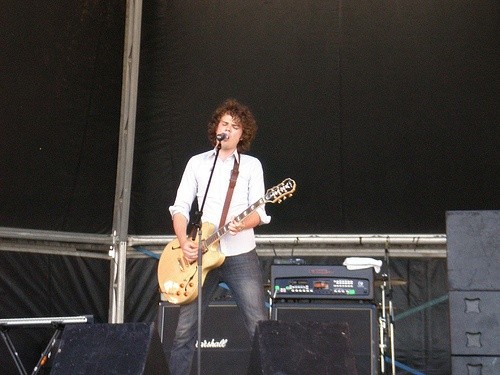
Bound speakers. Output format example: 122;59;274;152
247;319;357;375
159;301;270;375
51;323;171;375
271;304;379;375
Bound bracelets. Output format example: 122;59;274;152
239;220;246;232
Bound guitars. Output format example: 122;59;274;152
157;176;297;305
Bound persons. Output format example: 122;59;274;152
168;98;273;375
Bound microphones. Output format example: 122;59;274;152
217;133;227;142
